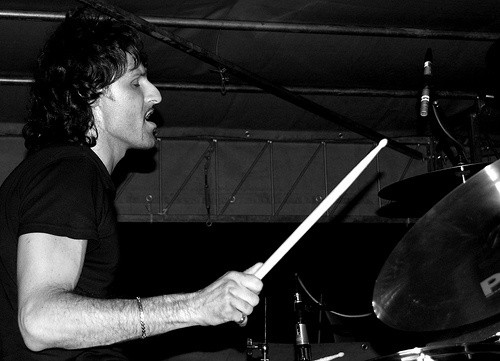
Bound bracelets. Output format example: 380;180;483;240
136;296;147;339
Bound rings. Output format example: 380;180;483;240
236;312;246;324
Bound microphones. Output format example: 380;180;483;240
420;48;434;117
293;292;312;361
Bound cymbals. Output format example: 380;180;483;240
371;158;500;332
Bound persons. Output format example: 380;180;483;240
0;9;263;361
